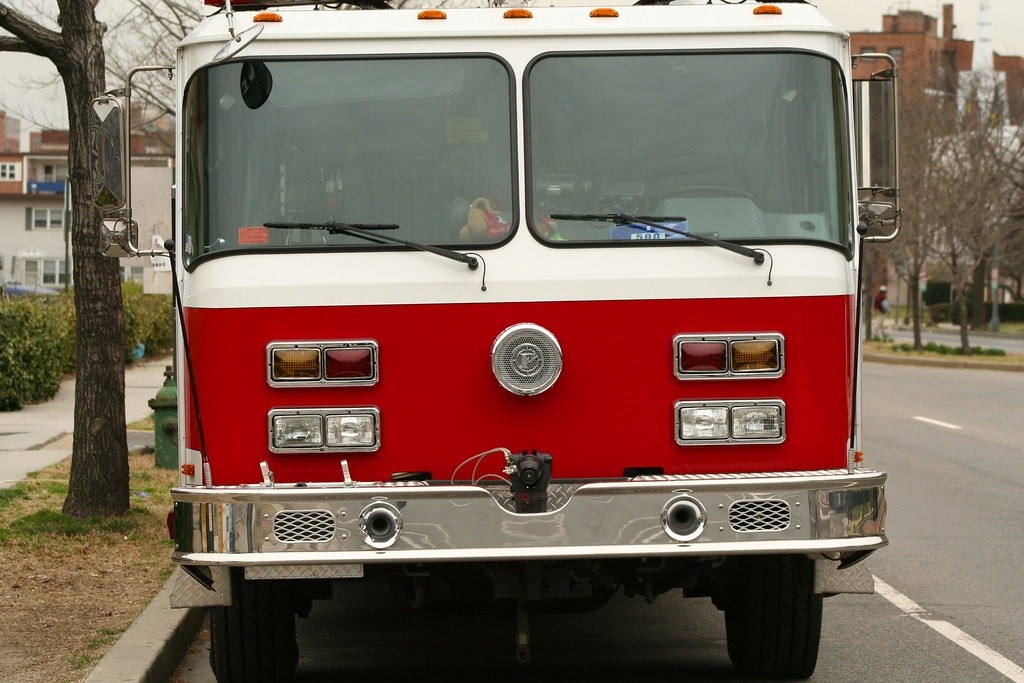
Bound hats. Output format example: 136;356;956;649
879;286;886;292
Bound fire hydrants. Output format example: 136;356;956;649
148;366;178;470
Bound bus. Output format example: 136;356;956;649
87;0;904;683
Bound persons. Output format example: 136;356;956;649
872;285;893;338
